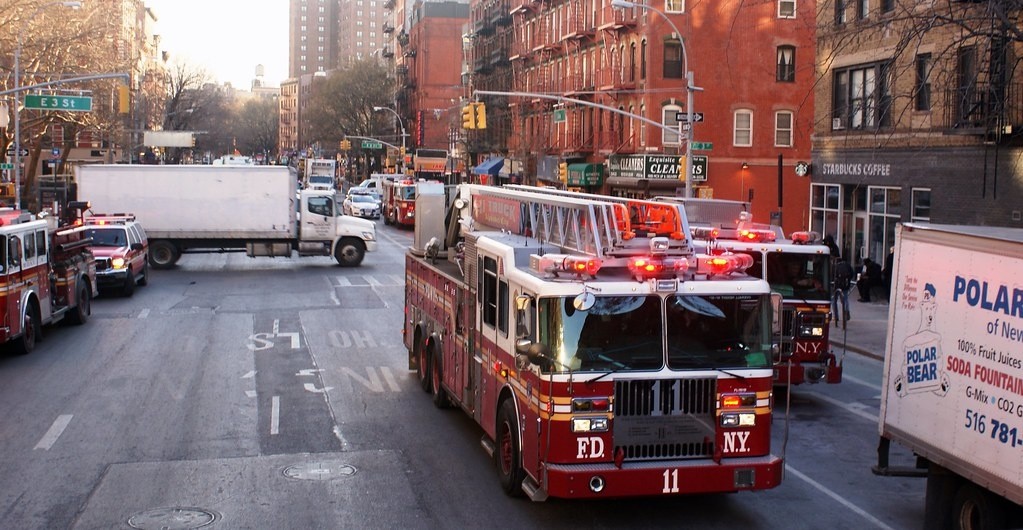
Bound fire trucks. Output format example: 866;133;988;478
0;208;99;354
403;182;843;502
382;178;416;231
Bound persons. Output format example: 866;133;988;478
828;249;853;321
857;258;881;302
822;235;840;258
881;246;894;301
672;305;709;341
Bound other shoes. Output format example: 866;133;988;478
858;297;870;302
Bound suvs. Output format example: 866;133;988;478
84;213;149;297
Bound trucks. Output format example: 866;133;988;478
361;174;403;197
871;223;1023;530
74;165;377;269
302;159;336;190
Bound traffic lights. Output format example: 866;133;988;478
556;162;567;184
401;147;405;156
462;106;476;129
679;155;687;184
340;141;352;150
476;105;486;129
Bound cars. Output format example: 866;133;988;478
343;187;382;219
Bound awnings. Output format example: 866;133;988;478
474;156;504;175
568;163;603;186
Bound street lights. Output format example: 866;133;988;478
14;3;81;211
374;107;406;175
611;0;693;200
